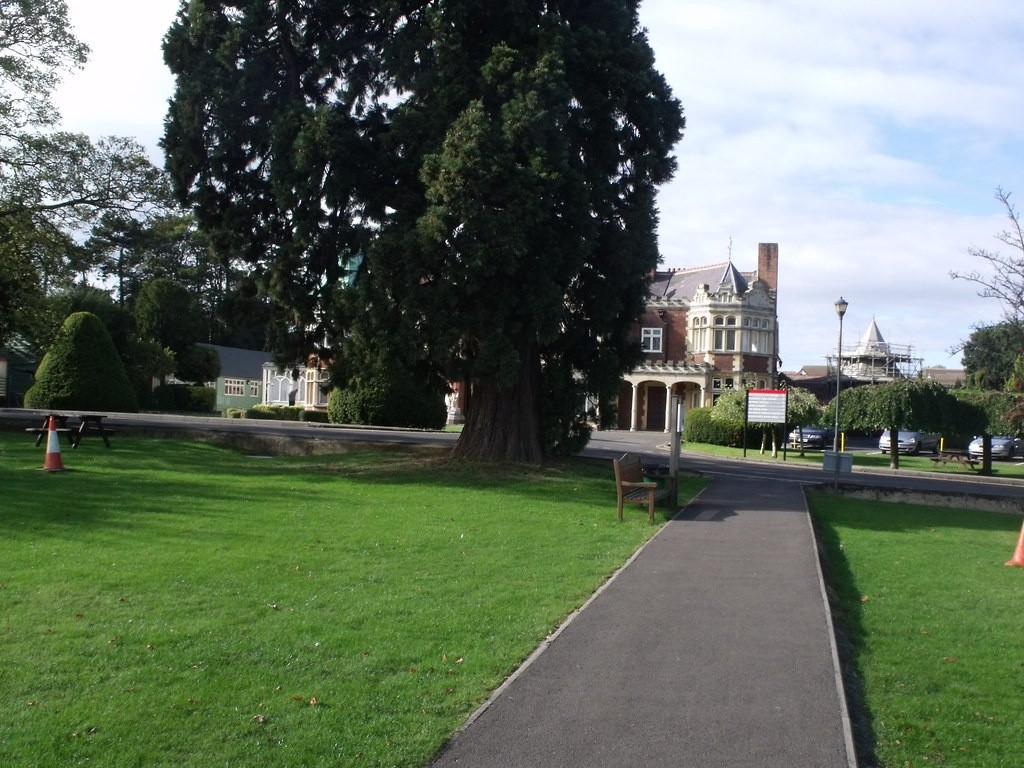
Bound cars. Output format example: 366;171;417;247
788;425;848;451
969;434;1024;462
879;427;945;456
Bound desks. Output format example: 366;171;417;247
34;413;111;449
932;451;974;472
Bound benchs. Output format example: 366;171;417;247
25;426;120;436
614;452;676;520
930;459;980;464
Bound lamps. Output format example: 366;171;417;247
659;309;665;317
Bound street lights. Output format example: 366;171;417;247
833;295;849;456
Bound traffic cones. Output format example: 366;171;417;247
42;416;65;471
1003;519;1024;568
